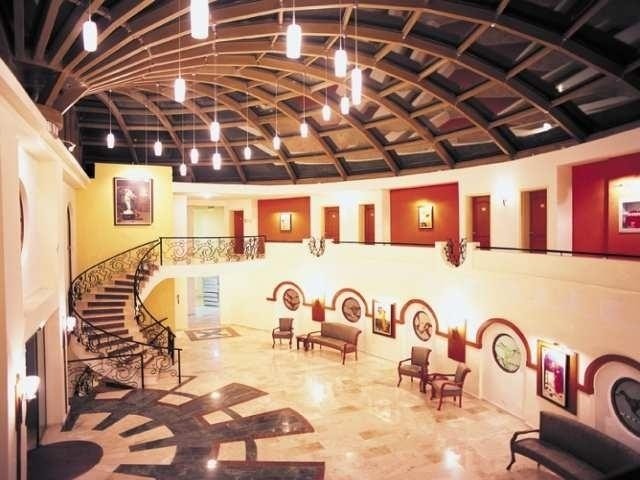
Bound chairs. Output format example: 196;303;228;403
272;317;294;349
397;345;432;392
430;360;472;411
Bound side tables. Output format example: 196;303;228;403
424;372;448;397
296;334;310;350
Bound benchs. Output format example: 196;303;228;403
307;320;362;365
506;411;640;479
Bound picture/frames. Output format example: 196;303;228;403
280;213;292;231
417;204;433;229
113;178;154;226
448;316;467;363
542;344;570;409
372;298;393;338
618;199;640;234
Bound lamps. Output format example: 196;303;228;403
341;81;350;116
334;7;346;76
211;34;221;143
287;3;302;60
301;75;308;137
174;3;185;102
323;60;330;121
213;146;222;169
108;91;114;148
274;84;280;150
17;374;42;402
154;85;161;156
352;5;362;103
180;101;187;177
194;3;209;39
85;5;97;52
191;85;198;163
245;89;250;159
67;316;77;333
